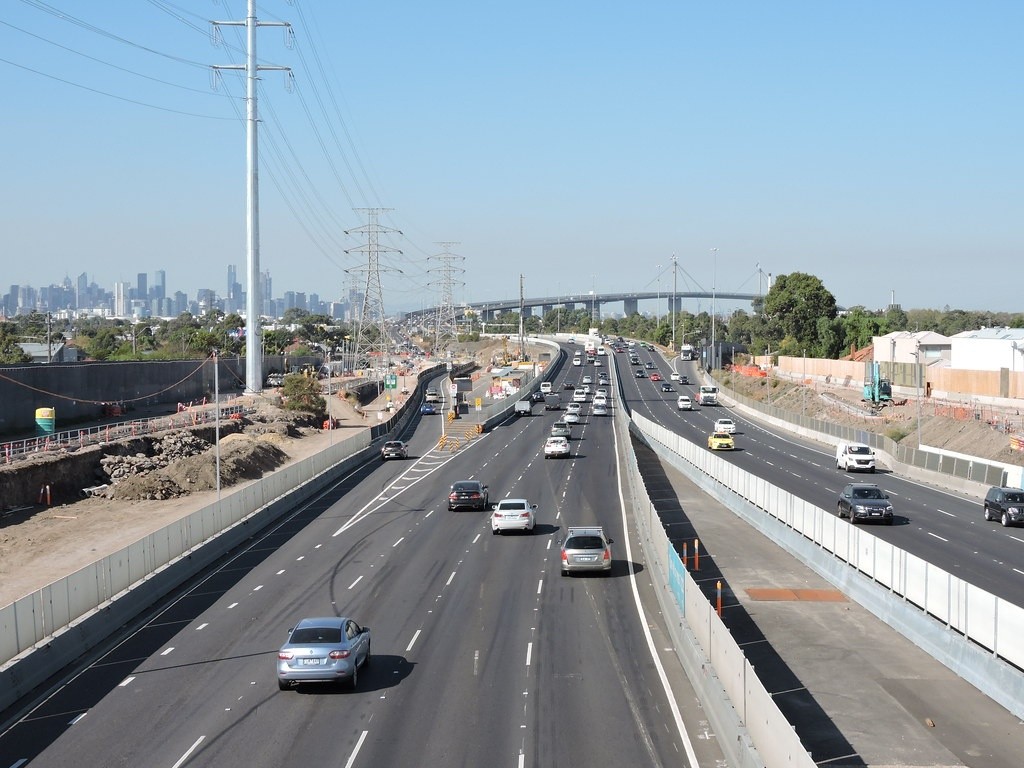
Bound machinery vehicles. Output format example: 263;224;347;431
862;361;892;409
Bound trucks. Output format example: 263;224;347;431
515;400;532;417
695;385;719;406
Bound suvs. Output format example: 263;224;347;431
551;422;572;438
837;483;893;525
558;525;615;576
425;387;439;402
544;436;571;459
983;486;1024;527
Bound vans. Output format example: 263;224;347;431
677;396;692;411
835;442;876;473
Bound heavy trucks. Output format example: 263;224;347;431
680;345;695;362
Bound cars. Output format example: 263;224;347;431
277;616;372;692
381;441;408;460
589;327;689;391
529;338;609;423
448;480;489;511
708;418;737;450
490;499;538;535
421;404;436;416
